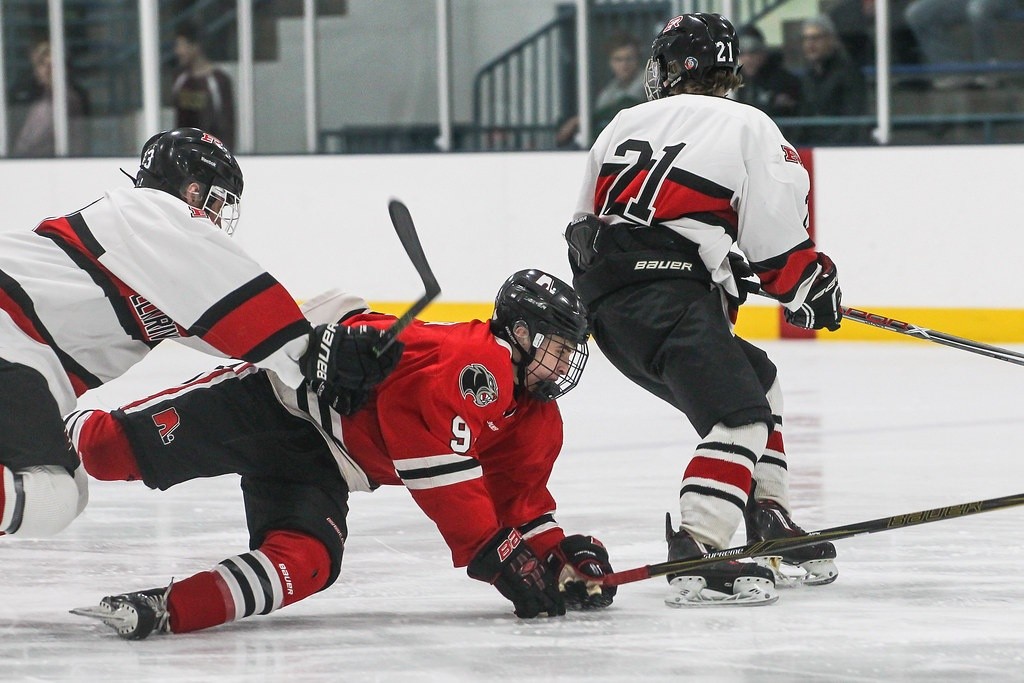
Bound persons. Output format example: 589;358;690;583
61;269;617;640
553;28;649;151
10;41;94;158
0;126;405;539
736;0;1007;146
161;20;240;156
566;12;843;609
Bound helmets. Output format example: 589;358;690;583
135;127;243;234
492;268;592;403
643;11;738;100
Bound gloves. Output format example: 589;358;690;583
543;534;617;610
467;525;566;618
299;321;403;415
782;256;844;330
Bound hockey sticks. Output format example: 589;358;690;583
370;197;445;361
600;492;1024;590
743;278;1024;369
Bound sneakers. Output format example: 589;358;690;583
665;511;779;606
744;477;837;588
70;575;177;641
